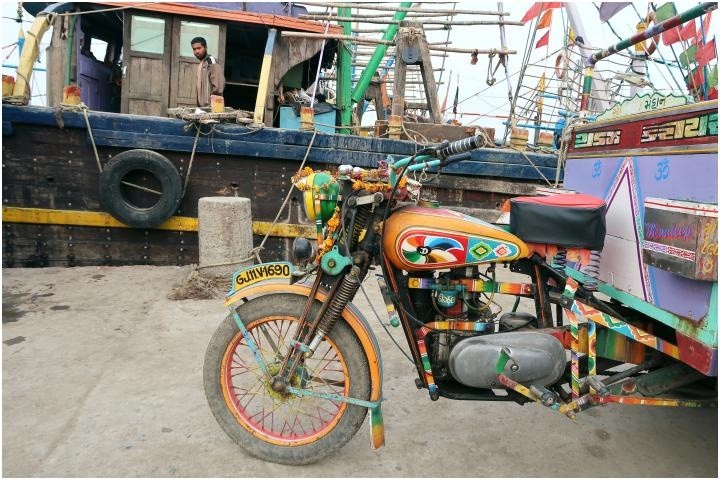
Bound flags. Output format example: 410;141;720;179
520;2;718;103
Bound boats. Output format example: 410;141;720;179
2;2;565;267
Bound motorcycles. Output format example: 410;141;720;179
200;2;718;467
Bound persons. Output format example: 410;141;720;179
191;37;227;112
